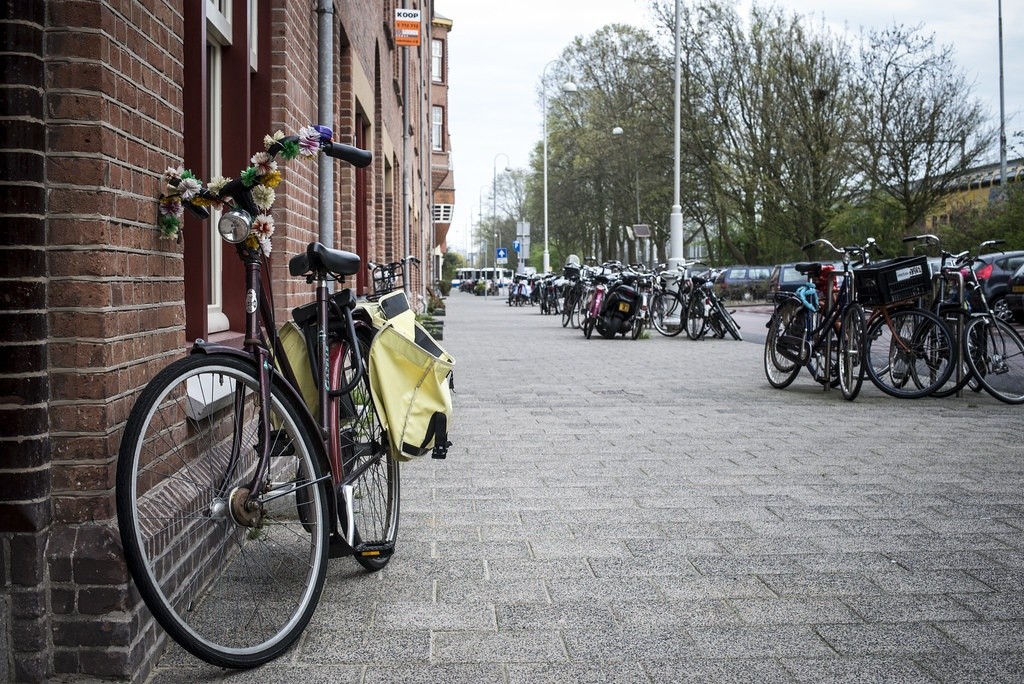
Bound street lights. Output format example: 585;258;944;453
541;58;578;275
477;153;512;299
612;109;641;223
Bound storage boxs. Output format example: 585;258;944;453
852;255;934;307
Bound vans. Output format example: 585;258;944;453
688;260;857;302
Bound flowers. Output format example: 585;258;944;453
157;126;321;258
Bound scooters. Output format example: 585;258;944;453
594;270;642;339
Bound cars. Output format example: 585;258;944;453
836;250;1024;325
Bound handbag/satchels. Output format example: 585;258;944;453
258;319;358;458
363;290;457;461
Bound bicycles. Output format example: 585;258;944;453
763;233;1024;405
506;255;742;342
116;125;424;669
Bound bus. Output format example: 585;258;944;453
452;267;513;288
